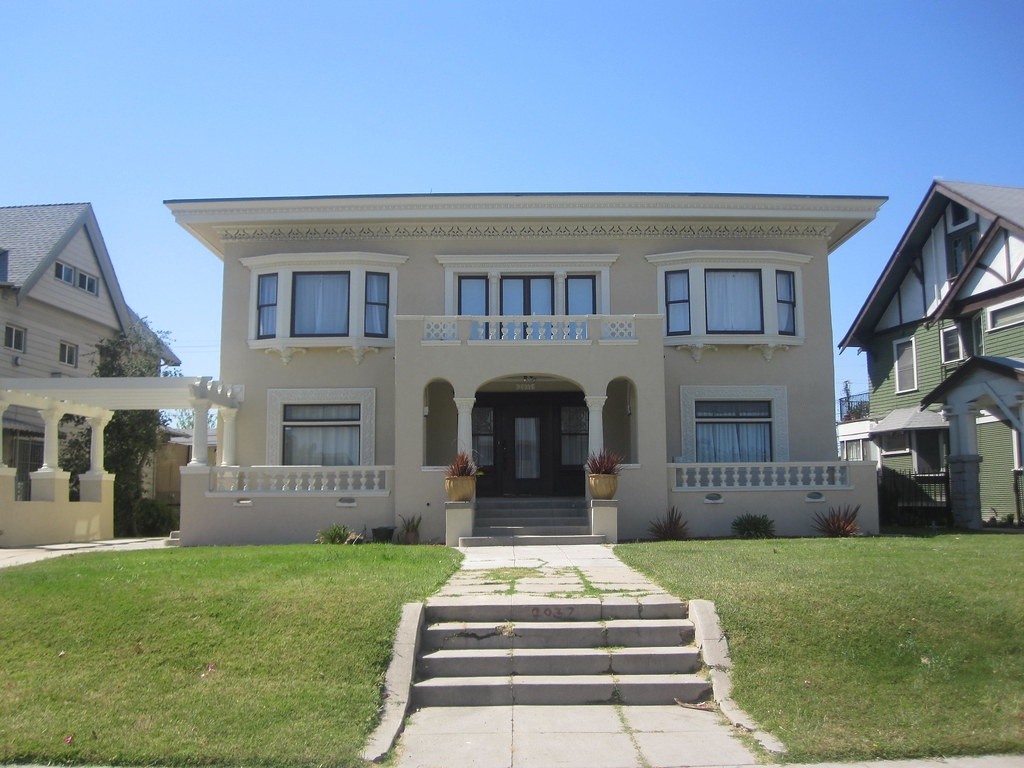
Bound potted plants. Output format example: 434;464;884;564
584;451;621;498
444;454;478;502
